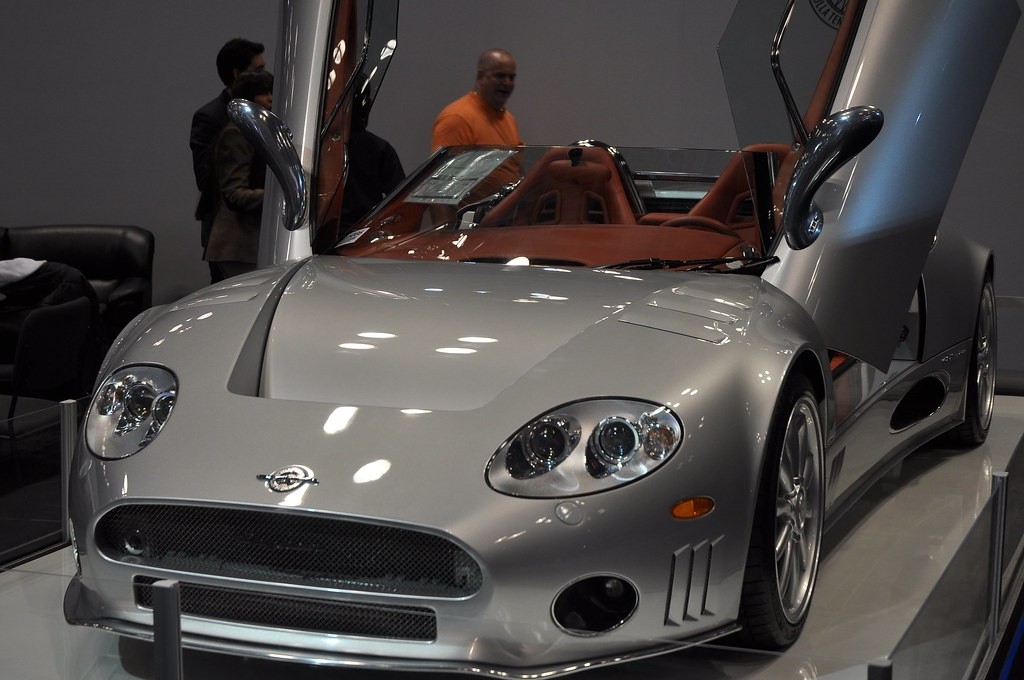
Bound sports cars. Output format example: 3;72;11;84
61;0;997;680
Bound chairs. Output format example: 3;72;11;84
0;224;154;456
480;147;646;228
687;144;796;251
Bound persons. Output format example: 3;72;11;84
311;72;406;255
204;64;274;279
189;38;267;286
431;49;526;226
